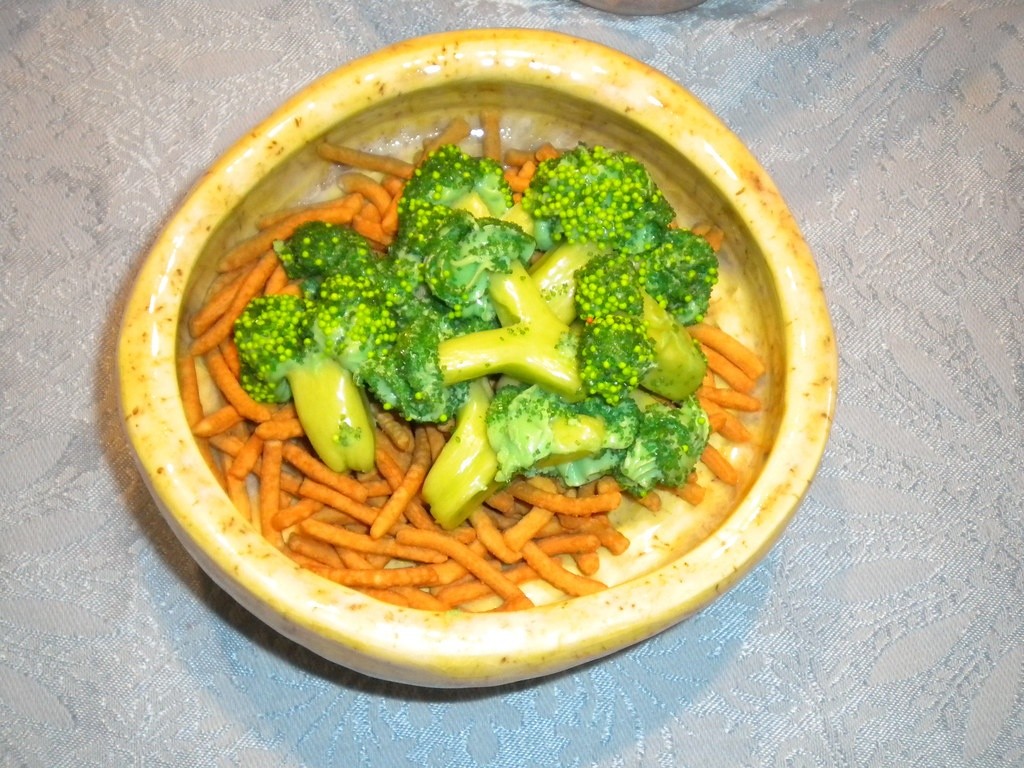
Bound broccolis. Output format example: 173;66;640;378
231;147;721;530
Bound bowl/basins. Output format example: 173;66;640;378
117;28;838;689
